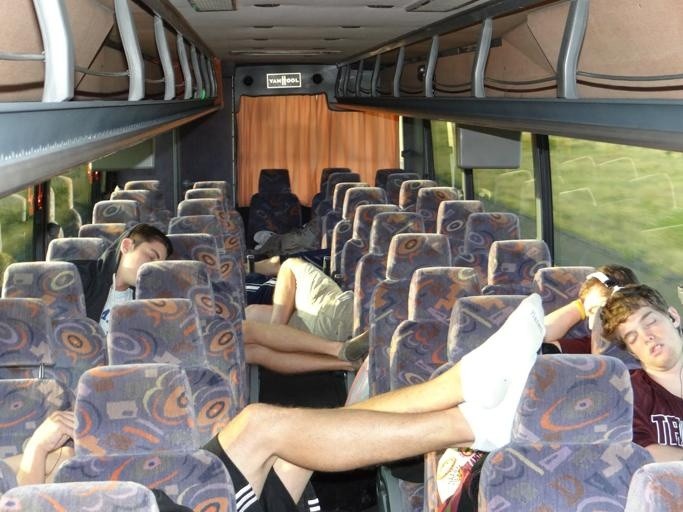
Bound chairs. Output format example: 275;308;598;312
0;166;259;511
311;167;682;511
245;166;303;262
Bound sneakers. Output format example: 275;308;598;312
336;331;369;362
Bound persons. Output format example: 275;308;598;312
435;262;640;512
599;282;683;464
1;292;547;512
